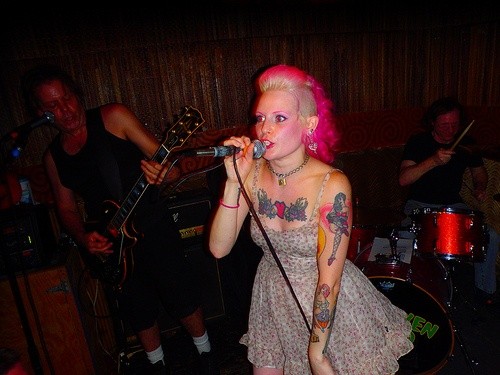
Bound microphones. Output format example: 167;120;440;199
179;140;264;158
0;110;54;143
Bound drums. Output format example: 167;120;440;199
341;223;379;262
354;243;459;375
408;206;495;261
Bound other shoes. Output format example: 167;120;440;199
192;346;219;375
150;360;172;375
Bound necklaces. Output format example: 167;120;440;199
266;153;309;186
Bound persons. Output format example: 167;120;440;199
21;64;219;375
209;64;414;375
399;96;500;307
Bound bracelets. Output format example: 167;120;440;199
220;199;239;208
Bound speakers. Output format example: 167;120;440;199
112;187;226;346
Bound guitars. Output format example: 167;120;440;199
78;105;207;293
